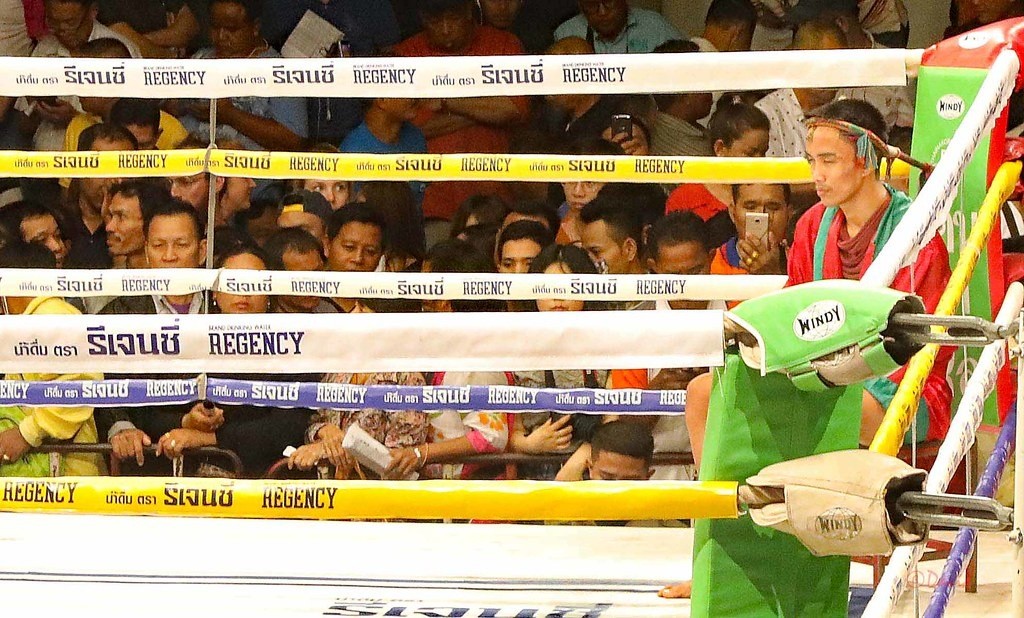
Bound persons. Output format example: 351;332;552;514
0;0;1024;528
662;99;956;599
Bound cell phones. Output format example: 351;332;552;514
611;114;633;141
745;212;769;251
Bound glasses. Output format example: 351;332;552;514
163;170;209;193
44;1;93;39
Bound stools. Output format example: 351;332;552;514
852;436;979;591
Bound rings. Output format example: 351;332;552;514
751;251;758;260
3;454;10;461
746;258;752;266
210;426;213;430
164;432;170;438
171;440;175;447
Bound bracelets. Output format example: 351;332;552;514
414;447;422;465
421;443;428;468
